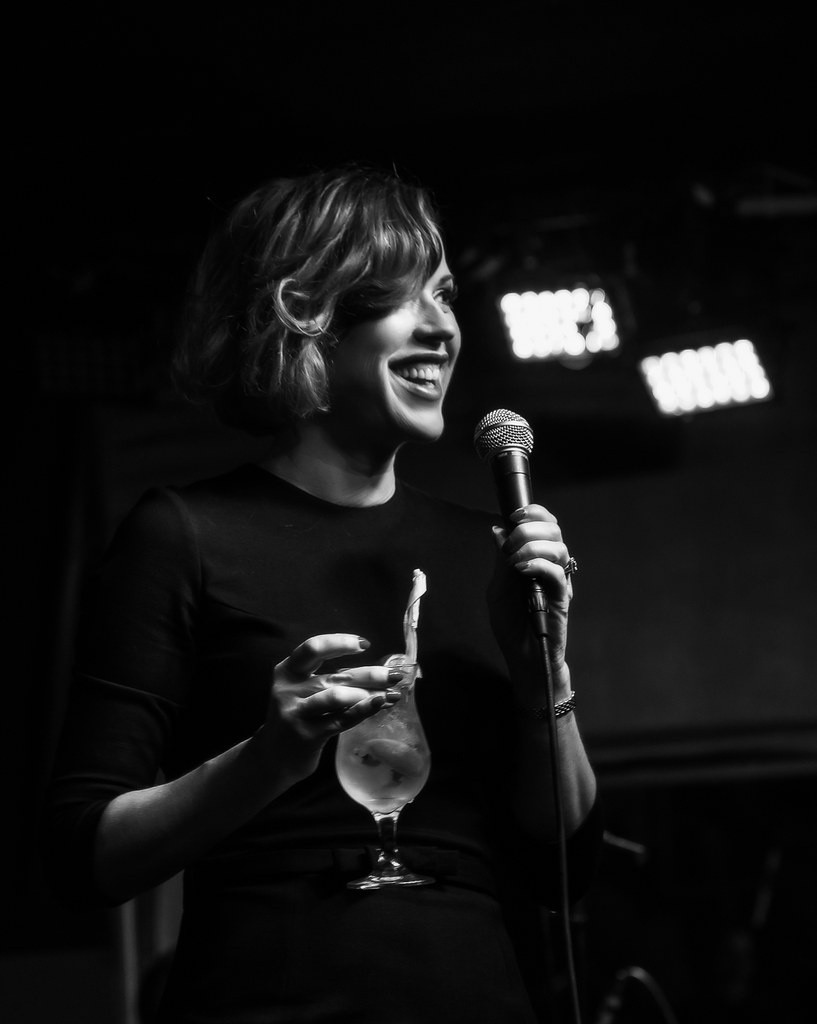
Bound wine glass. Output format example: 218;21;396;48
335;667;432;890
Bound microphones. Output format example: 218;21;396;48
474;410;551;638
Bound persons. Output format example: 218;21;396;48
42;165;598;1024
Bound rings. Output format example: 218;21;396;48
564;556;578;576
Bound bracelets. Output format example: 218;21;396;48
554;690;578;719
